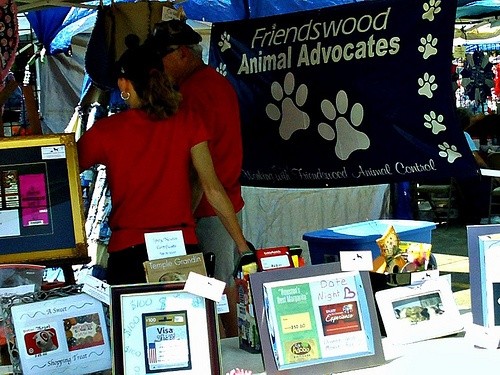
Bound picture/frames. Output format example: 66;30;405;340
0;132;88;265
375;276;465;348
467;224;500;326
0;283;113;375
248;261;386;375
109;281;224;375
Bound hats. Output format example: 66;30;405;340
144;20;202;45
108;44;164;80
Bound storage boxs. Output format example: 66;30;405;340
235;277;262;354
302;219;438;265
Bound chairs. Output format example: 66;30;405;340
411;179;454;230
475;168;500;223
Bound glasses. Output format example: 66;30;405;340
164;46;191;55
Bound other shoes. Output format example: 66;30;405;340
480;218;493;224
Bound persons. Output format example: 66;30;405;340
76;45;254;284
451;106;487;226
149;18;245;338
0;73;22;122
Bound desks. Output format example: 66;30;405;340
198;183;391;288
